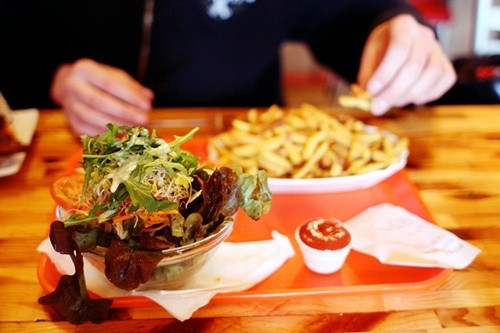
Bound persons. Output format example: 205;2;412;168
1;0;460;140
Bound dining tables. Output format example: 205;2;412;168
0;102;500;331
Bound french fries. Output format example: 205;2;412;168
209;84;410;177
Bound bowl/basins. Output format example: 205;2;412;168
294;218;352;274
56;196;240;292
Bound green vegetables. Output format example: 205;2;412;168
37;121;273;326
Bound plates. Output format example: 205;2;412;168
265;125;409;194
1;107;41;177
